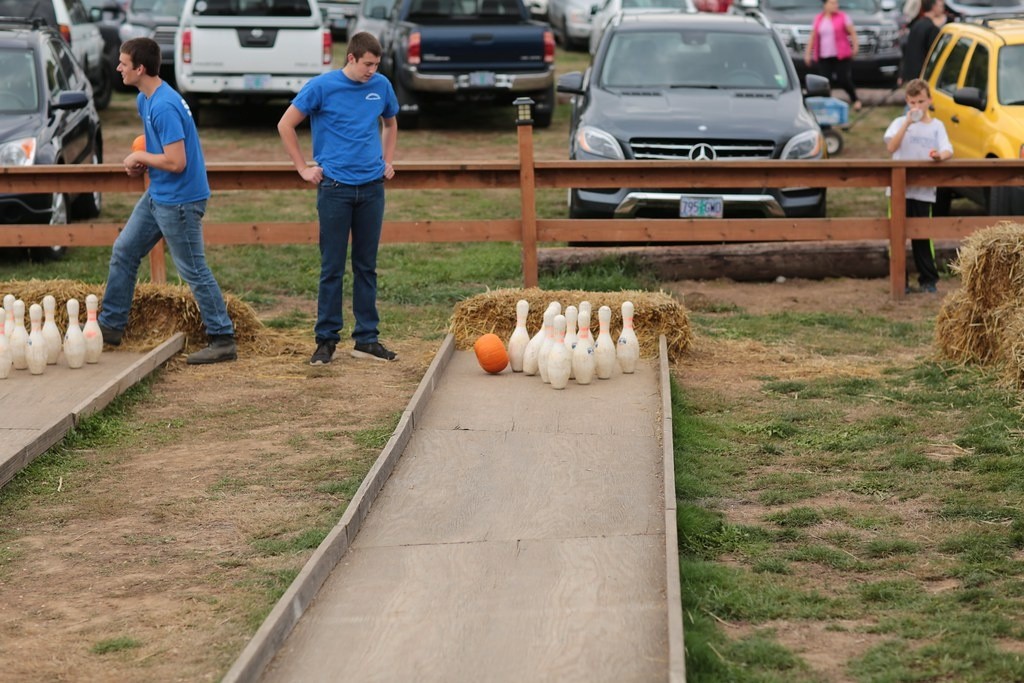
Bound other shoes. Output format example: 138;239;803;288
903;284;935;295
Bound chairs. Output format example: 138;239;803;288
607;38;670;82
470;0;503;19
410;0;447;19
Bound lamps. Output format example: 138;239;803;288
512;97;535;125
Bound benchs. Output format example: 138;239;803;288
198;0;304;15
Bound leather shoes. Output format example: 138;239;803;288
186;339;238;364
65;320;123;346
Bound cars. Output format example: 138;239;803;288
549;1;605;44
318;1;392;46
904;0;1024;33
0;1;113;113
84;0;183;89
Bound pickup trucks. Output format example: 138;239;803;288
379;1;555;131
173;0;333;125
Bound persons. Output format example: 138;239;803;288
898;0;946;86
98;37;238;365
805;0;864;113
882;80;954;292
278;31;399;363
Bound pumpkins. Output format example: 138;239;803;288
473;333;508;373
132;135;146;152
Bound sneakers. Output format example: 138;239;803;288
309;340;340;365
351;340;398;361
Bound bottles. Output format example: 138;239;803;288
910;109;924;122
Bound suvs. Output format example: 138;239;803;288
919;12;1024;216
730;1;902;90
557;8;830;247
0;16;102;260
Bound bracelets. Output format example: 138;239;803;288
299;166;308;173
